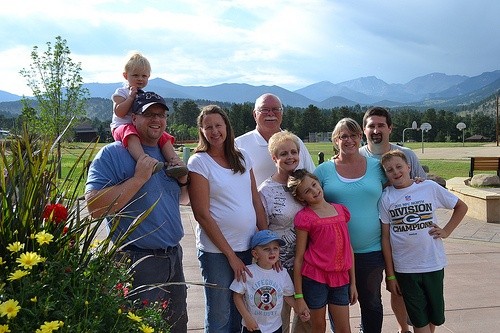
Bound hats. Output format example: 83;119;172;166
133;91;169;115
251;229;286;249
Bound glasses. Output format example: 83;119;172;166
259;107;283;114
142;112;167;118
340;134;359;140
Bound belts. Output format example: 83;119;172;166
124;245;180;255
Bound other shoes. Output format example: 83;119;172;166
165;165;188;178
153;162;164;175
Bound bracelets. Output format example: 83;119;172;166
180;179;189;187
294;294;303;298
388;276;395;280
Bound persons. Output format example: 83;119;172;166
256;129;308;333
287;169;358;333
110;53;188;179
312;118;424;333
377;149;469;333
187;105;269;333
85;92;191;333
234;92;315;333
358;106;428;333
229;230;310;333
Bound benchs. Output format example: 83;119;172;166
469;157;500;178
